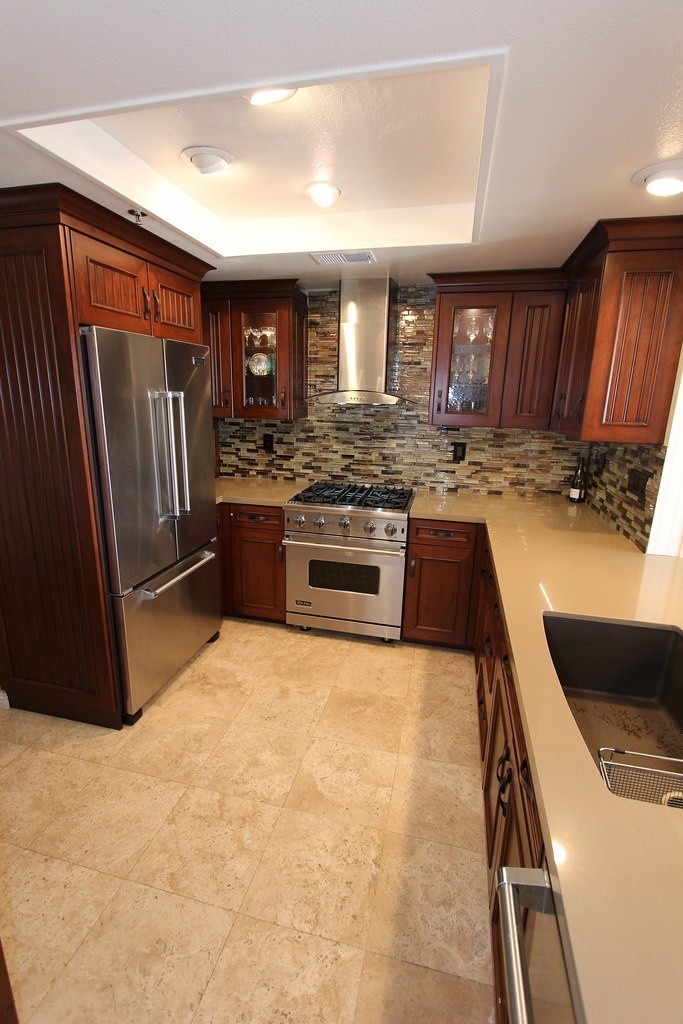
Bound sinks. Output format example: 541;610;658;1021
542;609;683;811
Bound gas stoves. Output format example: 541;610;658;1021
289;480;415;513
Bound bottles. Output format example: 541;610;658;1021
570;457;586;503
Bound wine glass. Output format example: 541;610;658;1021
244;326;252;346
251;328;263;347
245;356;250;376
261;326;276;347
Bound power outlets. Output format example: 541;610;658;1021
263;433;274;451
627;468;647;496
453;442;467;461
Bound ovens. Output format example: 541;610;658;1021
281;530;406;643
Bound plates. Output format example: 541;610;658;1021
249;353;268;376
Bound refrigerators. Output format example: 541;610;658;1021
78;325;223;726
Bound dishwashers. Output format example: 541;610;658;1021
495;849;584;1024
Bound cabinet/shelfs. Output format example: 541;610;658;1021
472;525;507;762
482;655;544;929
425;267;565;431
70;230;204;344
228;504;286;624
548;214;683;445
401;518;478;651
200;278;308;421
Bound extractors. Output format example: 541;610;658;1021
302;277;417;408
